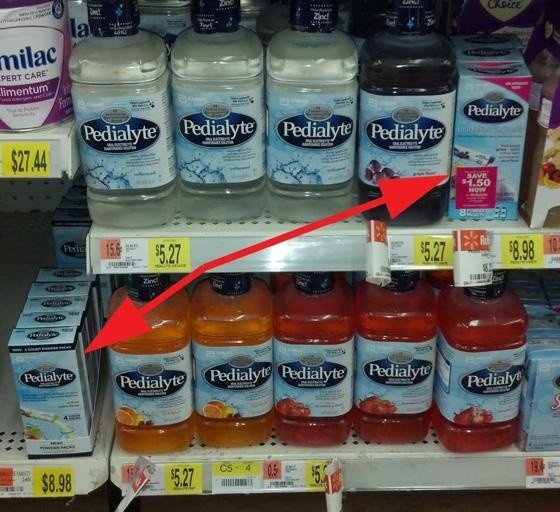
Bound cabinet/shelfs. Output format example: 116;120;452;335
0;122;560;500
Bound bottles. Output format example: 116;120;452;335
68;0;457;229
518;40;560;229
106;271;526;454
